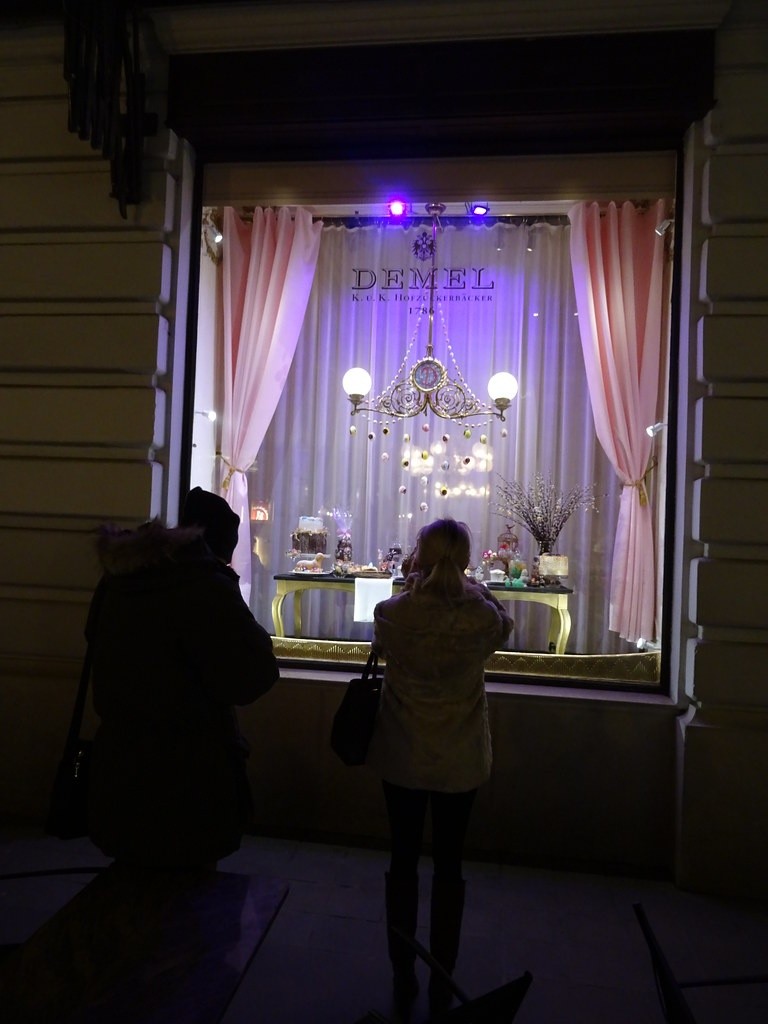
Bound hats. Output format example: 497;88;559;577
181;486;240;553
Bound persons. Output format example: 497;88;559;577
79;487;281;1019
371;516;514;1020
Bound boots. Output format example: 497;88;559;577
384;871;420;1004
427;874;466;1014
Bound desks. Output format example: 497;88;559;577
272;572;573;655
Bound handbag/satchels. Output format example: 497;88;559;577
42;745;101;839
329;648;383;768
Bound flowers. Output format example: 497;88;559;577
485;471;610;554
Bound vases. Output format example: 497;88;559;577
533;554;569;584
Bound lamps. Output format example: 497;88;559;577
341;202;518;512
